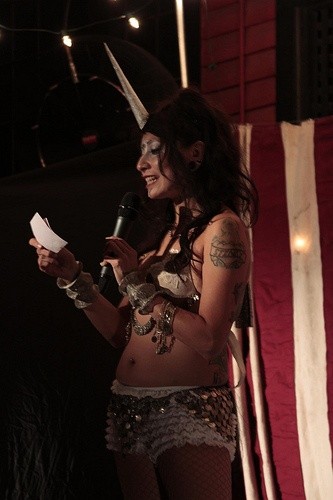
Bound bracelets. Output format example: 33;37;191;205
118;270;177;354
55;261;99;308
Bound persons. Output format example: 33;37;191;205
27;87;258;500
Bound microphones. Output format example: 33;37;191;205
94;194;141;296
